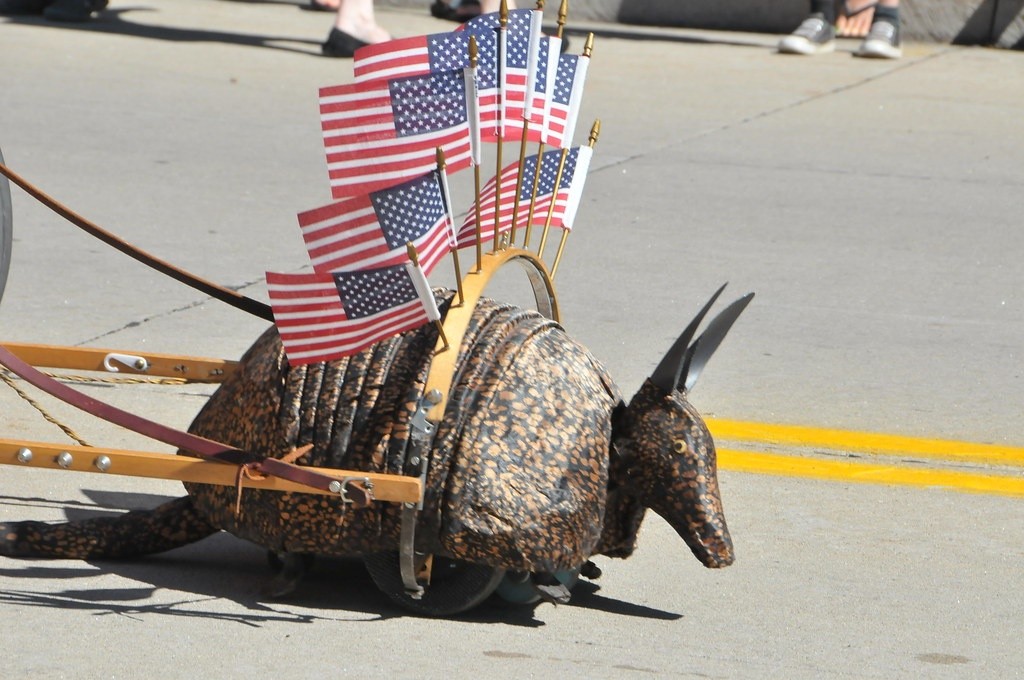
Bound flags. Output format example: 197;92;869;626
482;36;562;144
450;146;596;252
297;171;458;277
548;52;591;148
355;30;505;142
452;9;543;120
268;265;438;369
318;70;480;199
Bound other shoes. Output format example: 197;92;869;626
327;28;397;55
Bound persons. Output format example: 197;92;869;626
431;1;568;52
322;0;395;57
779;0;901;60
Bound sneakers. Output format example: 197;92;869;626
862;18;902;57
778;13;834;54
